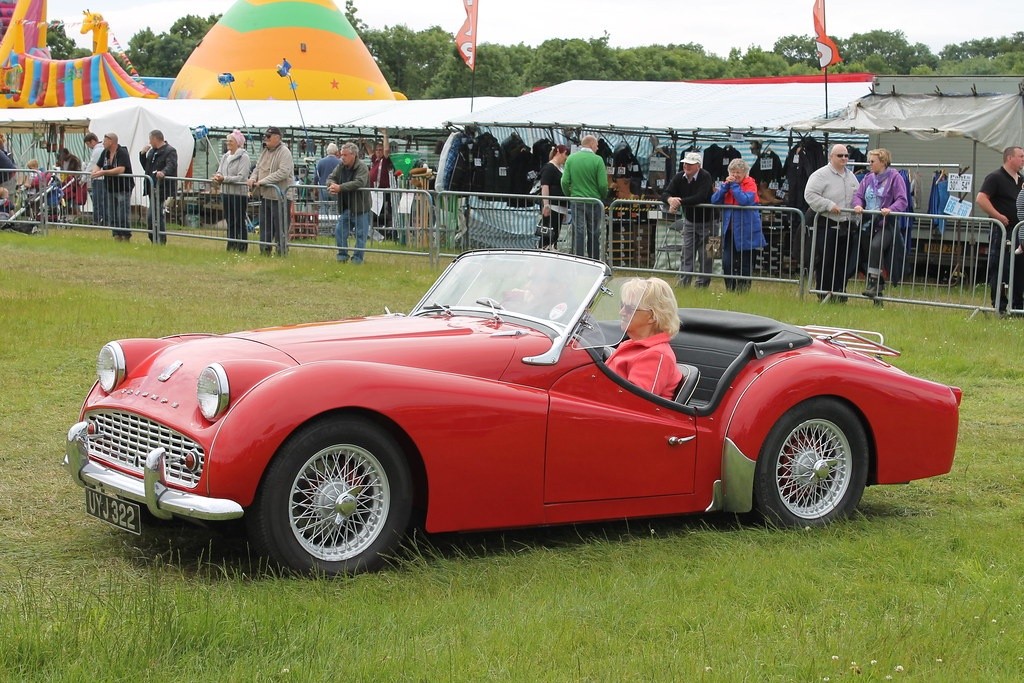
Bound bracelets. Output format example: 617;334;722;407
544;204;549;208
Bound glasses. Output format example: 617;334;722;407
104;134;112;140
620;301;647;311
833;153;849;158
264;134;271;139
868;159;880;163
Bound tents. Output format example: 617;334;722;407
88;80;874;168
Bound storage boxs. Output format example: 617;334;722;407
603;195;666;266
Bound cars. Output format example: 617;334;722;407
63;246;958;583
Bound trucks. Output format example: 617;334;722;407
865;72;1024;284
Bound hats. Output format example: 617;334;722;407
679;152;702;165
261;126;282;137
231;129;245;146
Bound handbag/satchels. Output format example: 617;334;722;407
529;165;556;195
535;213;555;239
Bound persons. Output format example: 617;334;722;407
0;126;397;264
540;136;1024;317
604;278;682;401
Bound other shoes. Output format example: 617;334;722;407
862;276;885;308
114;234;130;243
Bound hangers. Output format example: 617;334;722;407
466;121;805;161
856;163;948;185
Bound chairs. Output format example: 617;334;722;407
599;321;750;403
288;196;319;240
651;218;683;274
